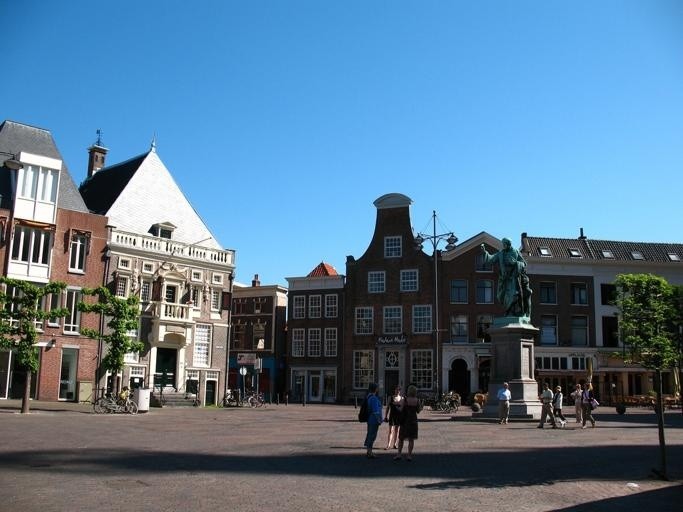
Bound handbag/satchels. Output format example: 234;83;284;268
589;399;599;409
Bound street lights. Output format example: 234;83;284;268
411;209;460;413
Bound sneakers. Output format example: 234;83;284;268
363;442;413;462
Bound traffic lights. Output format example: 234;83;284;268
220;292;231;309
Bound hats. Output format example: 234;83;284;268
554;386;561;391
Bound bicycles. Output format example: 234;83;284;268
415;388;461;414
224;387;264;408
91;385;141;417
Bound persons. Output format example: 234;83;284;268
554;386;568;424
482;238;527;316
497;382;511;424
364;382;383;458
392;385;420;461
384;385;403;449
571;384;584;424
537;383;557;429
581;383;597;429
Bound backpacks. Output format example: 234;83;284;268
359;393;373;422
